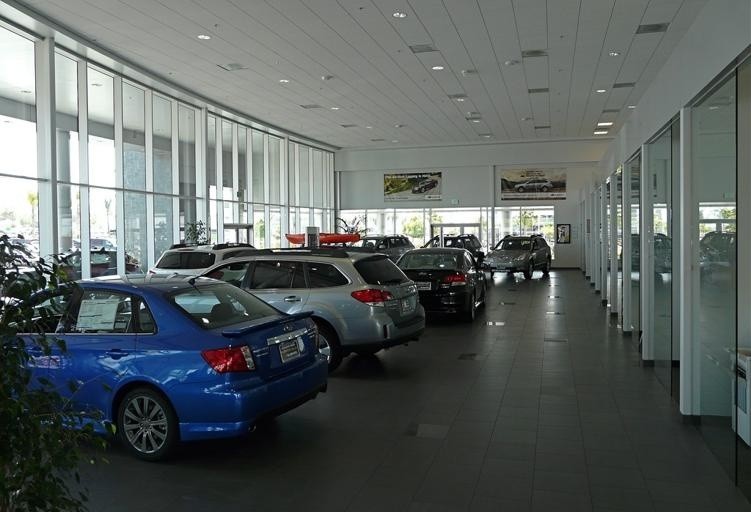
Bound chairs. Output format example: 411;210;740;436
209;304;233;321
127;309;154;335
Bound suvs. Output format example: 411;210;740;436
44;237;144;286
515;178;553;192
0;238;40;269
147;242;426;372
412;179;438;193
346;234;551;279
600;232;736;293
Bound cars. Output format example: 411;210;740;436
396;247;488;323
11;273;329;461
